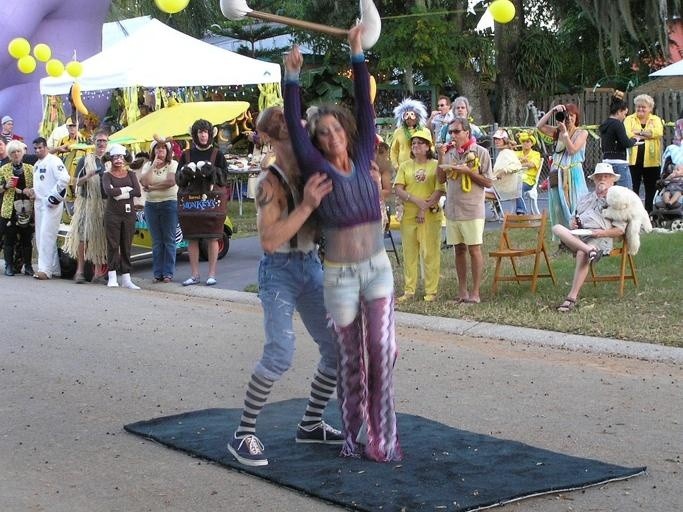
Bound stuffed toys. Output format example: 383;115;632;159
602;185;652;254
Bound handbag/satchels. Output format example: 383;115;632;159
550;170;558;187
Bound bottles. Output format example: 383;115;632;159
575;209;583;229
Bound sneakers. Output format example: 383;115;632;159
4;263;217;290
397;294;413;302
423;294;437;301
226;430;268;466
295;420;345;444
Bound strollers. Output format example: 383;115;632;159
648;144;682;232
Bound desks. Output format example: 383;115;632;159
226;157;263;215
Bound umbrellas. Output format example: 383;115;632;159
648;59;683;77
109;99;250;144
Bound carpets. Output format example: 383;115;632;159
124;397;649;512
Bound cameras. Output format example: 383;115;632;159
555;109;567;122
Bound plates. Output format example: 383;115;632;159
438;163;467;170
569;229;593;236
633;141;645;146
248;168;262;172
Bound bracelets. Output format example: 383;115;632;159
562;132;568;137
639;132;643;135
406;194;409;201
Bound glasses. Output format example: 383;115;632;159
437;104;444;107
448;129;462;135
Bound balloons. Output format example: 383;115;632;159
8;37;81;77
488;0;515;24
155;0;190;14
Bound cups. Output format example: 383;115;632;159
11;176;19;188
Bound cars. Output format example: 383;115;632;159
56;146;233;277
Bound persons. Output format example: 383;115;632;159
370;134;392;235
394;129;447;304
552;163;626;312
426;96;543;217
437;118;493;303
390;97;432;169
598;95;647;189
623;94;663;215
227;107;347;468
536;103;589;258
282;21;402;464
1;117;227;289
663;162;683;208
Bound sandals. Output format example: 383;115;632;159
556;297;577;314
588;246;604;264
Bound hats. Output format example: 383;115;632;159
150;134;172;151
66;117;79;126
519;129;537;145
587;162;621;183
101;144;132;164
2;115;14;124
410;128;433;146
492;130;508;144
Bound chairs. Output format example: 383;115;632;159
523;157;545;215
383;205;401;265
573;232;639;297
488;207;556;294
487;171;528;220
